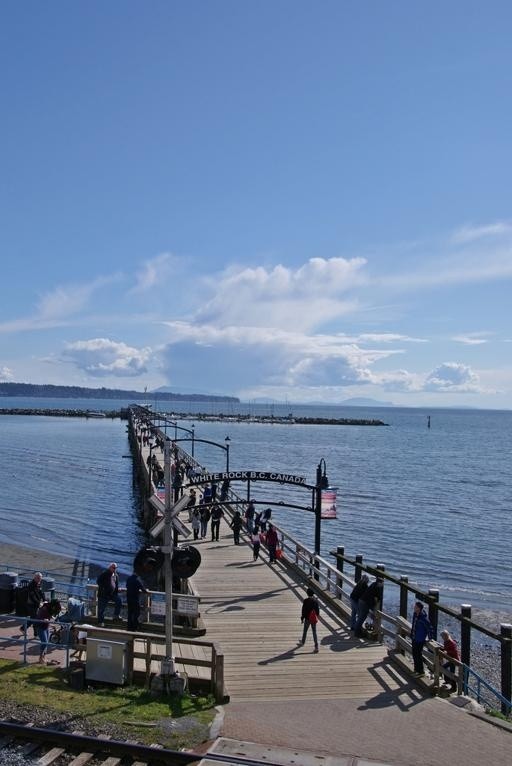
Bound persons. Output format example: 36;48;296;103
94;562;123;628
34;598;62;664
131;407;280;564
125;569;152;633
439;630;459;693
298;587;320;653
19;572;48;640
350;574;369;632
354;577;384;638
407;602;434;680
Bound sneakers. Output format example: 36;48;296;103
409;671;425;679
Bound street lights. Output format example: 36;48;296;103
158;412;177;443
314;458;329;581
224;436;231;472
191;423;196;457
148;434;155;490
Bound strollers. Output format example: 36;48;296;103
40;598;85;627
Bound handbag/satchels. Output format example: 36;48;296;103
259;534;266;544
276;549;283;559
309;610;318;624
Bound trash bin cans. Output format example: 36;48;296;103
40;576;57;603
16;578;33;617
0;571;18;614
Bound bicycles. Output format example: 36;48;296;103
49;618;79;645
